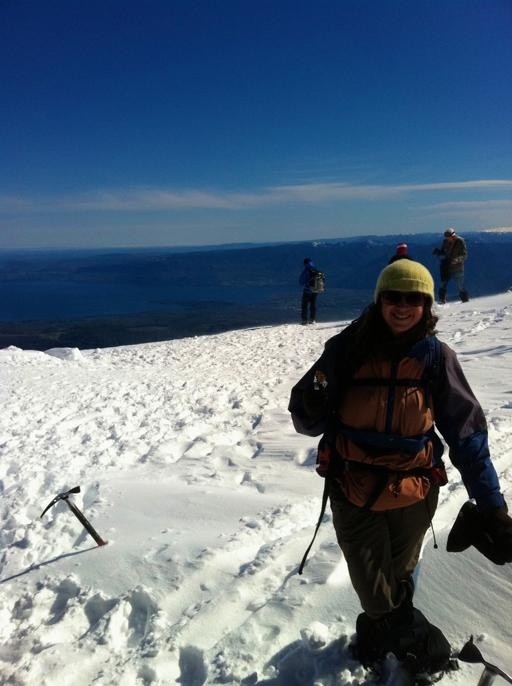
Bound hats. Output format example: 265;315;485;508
374;259;435;304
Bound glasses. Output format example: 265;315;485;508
381;291;425;306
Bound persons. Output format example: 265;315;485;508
431;228;469;304
388;242;413;266
298;257;317;326
288;258;510;686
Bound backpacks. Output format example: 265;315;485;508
304;268;325;294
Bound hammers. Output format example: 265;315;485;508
39;486;107;547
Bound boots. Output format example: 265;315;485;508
438;299;446;304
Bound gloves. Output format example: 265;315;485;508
296;380;333;419
447;496;512;565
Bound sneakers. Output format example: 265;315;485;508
301;320;316;325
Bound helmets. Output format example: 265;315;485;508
444;228;456;238
397;243;407;256
